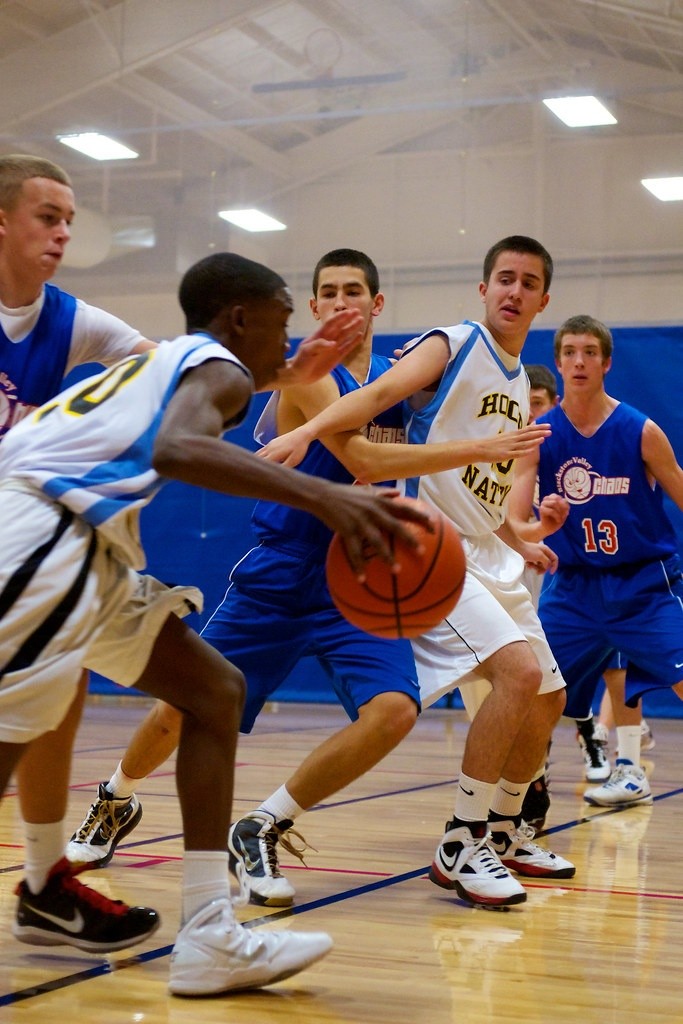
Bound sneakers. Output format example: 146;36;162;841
428;820;527;908
489;817;576;878
227;810;318;907
615;723;654;754
583;763;653;807
66;780;142;869
167;899;333;994
575;726;607;748
13;856;161;954
580;741;612;781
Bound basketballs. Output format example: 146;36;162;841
323;495;468;642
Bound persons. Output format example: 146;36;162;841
507;314;683;831
67;248;420;908
0;157;366;956
255;236;576;907
2;252;433;999
